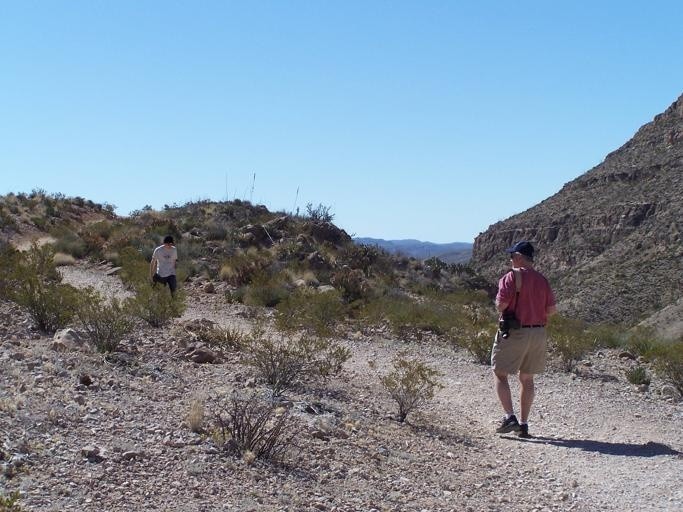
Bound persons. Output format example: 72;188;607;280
148;235;180;297
488;240;559;440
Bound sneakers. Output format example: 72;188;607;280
514;424;528;437
496;415;519;433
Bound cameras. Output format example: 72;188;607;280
498;310;520;339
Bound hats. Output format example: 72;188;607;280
506;241;533;257
163;235;174;244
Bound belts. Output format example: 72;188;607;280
521;325;546;327
